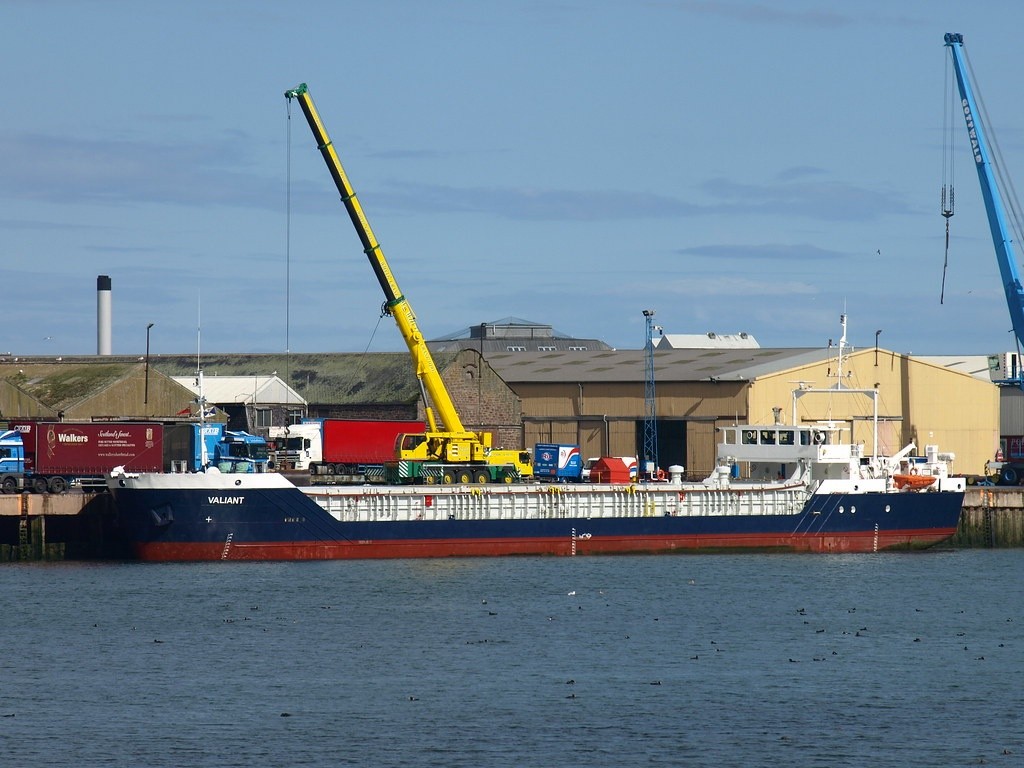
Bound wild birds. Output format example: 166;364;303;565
568;589;575;596
56;356;63;361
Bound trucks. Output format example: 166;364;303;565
165;421;276;473
533;442;585;484
0;419;164;495
580;456;637;483
977;459;1024;487
274;417;426;476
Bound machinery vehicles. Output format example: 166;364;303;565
938;32;1024;486
281;81;536;484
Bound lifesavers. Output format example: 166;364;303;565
657;470;665;481
747;432;753;440
910;468;918;475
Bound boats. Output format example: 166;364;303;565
66;316;968;566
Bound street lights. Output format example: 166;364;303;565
144;323;155;404
